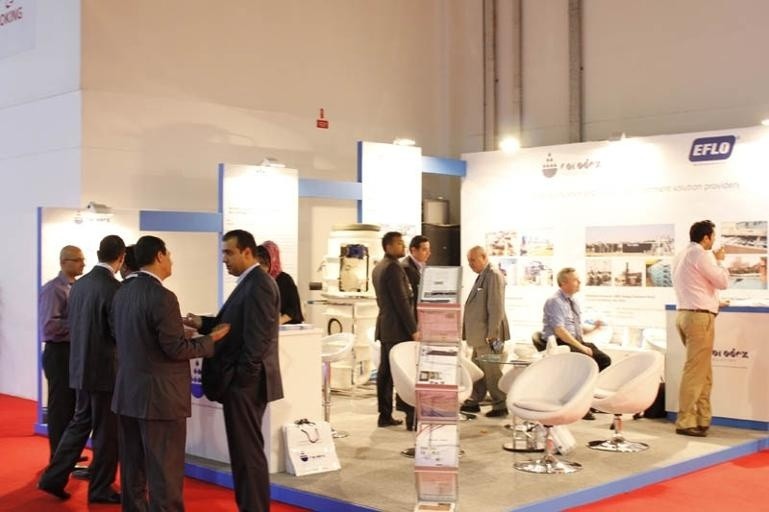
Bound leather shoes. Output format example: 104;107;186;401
460;405;480;413
407;426;416;431
698;425;709;431
582;412;595;420
590;408;604;413
90;493;121;503
378;417;403;427
485;408;508;417
73;469;91;478
78;457;89;462
38;477;72;500
72;465;88;471
675;428;705;436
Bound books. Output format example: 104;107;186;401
417;471;458;502
415;424;455;466
420;312;459;343
417;345;458;387
420;266;459;305
419;391;456;418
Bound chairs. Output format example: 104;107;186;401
639;326;667;383
504;351;600;474
586;349;666;454
497;344;571;455
321;332;355;440
387;340;475;459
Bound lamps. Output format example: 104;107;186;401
608;130;627;143
86;202;114;219
260;157;288;169
393;138;416;147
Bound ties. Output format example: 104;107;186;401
567;298;583;344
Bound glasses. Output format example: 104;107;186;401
63;257;85;262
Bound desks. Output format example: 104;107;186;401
667;302;769;431
475;352;530;365
185;325;322;476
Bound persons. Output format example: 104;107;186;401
109;235;231;512
670;219;732;438
461;244;511;417
370;231;420;430
542;268;612;421
251;241;304;326
117;243;144;279
36;235;128;505
179;229;285;512
399;235;431;327
40;245;90;463
